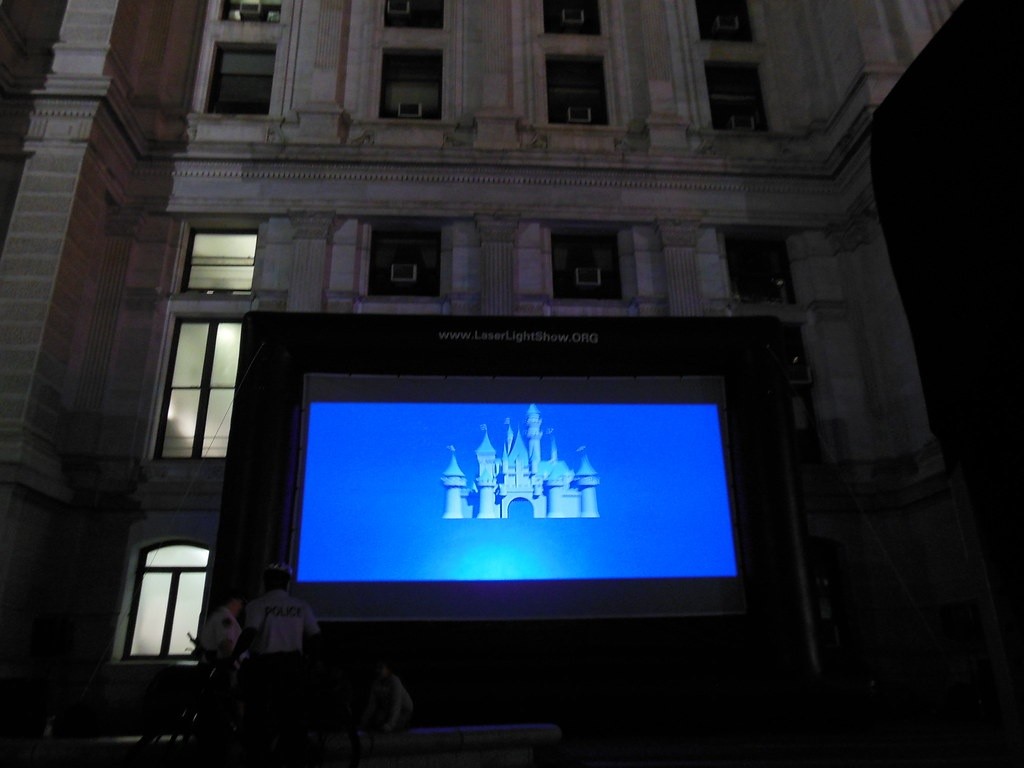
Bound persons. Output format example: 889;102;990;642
194;569;414;768
